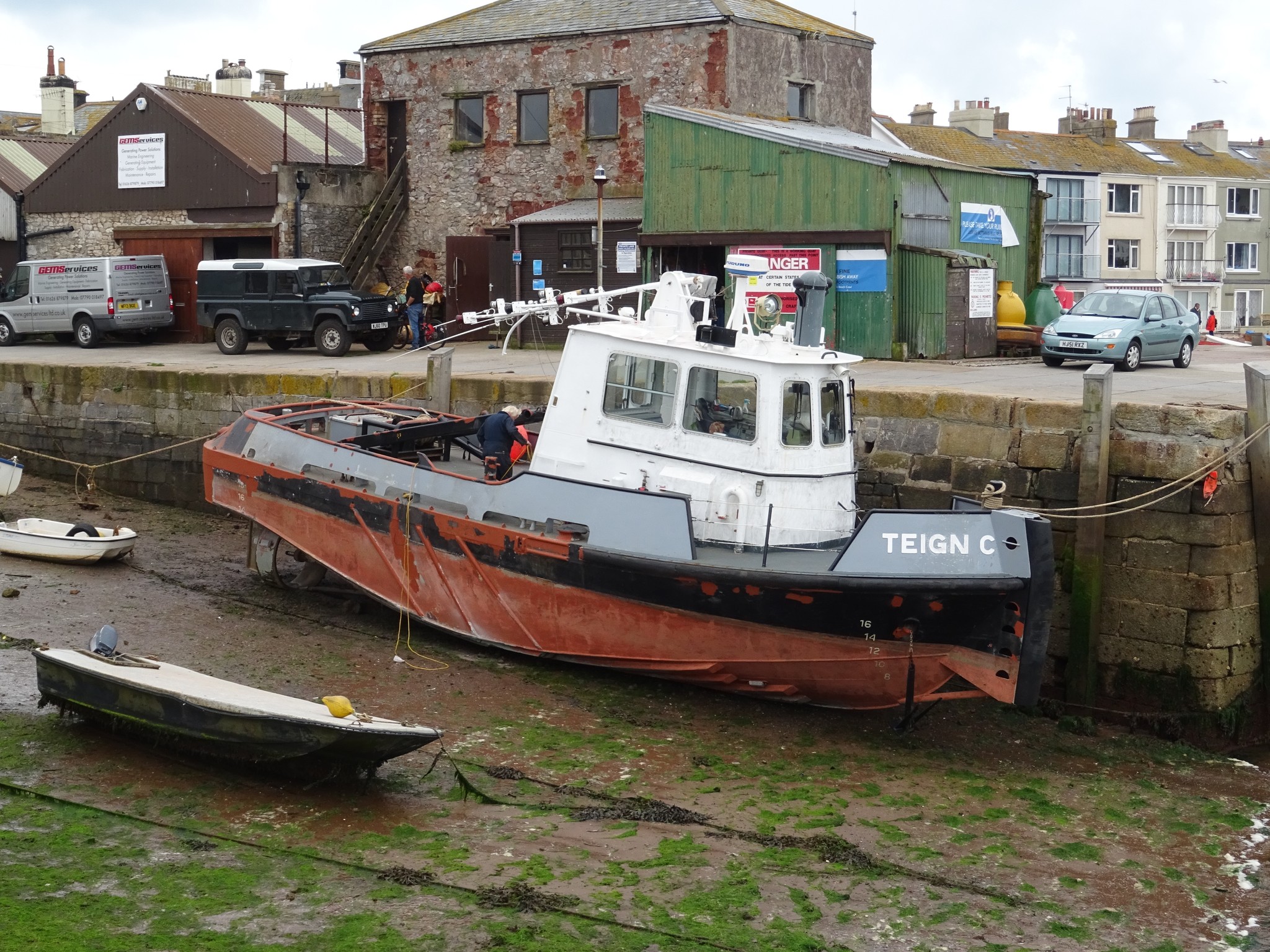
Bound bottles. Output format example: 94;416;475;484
715;397;720;411
1023;281;1063;328
996;280;1026;327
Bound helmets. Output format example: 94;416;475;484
425;282;442;293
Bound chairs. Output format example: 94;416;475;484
695;398;733;434
275;273;294;295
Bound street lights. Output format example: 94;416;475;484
591;166;611;324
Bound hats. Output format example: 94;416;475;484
1210;310;1214;314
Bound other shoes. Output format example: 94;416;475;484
420;345;428;349
405;346;420;351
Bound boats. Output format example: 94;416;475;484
0;518;140;566
0;458;25;498
31;646;445;779
200;270;1058;714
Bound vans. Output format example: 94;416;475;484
0;254;177;348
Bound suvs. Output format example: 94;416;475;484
196;257;404;358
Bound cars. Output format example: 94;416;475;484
1040;288;1201;372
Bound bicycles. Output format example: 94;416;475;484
393;302;448;351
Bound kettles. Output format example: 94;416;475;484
729;406;743;422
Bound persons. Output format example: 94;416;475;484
1189;303;1202;331
476;405;531;482
1205;309;1215;336
402;266;427;352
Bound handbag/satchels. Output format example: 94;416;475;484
1215;318;1217;327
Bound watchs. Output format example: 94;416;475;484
406;303;409;308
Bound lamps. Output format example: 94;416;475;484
753;294;783;333
756;481;764;497
